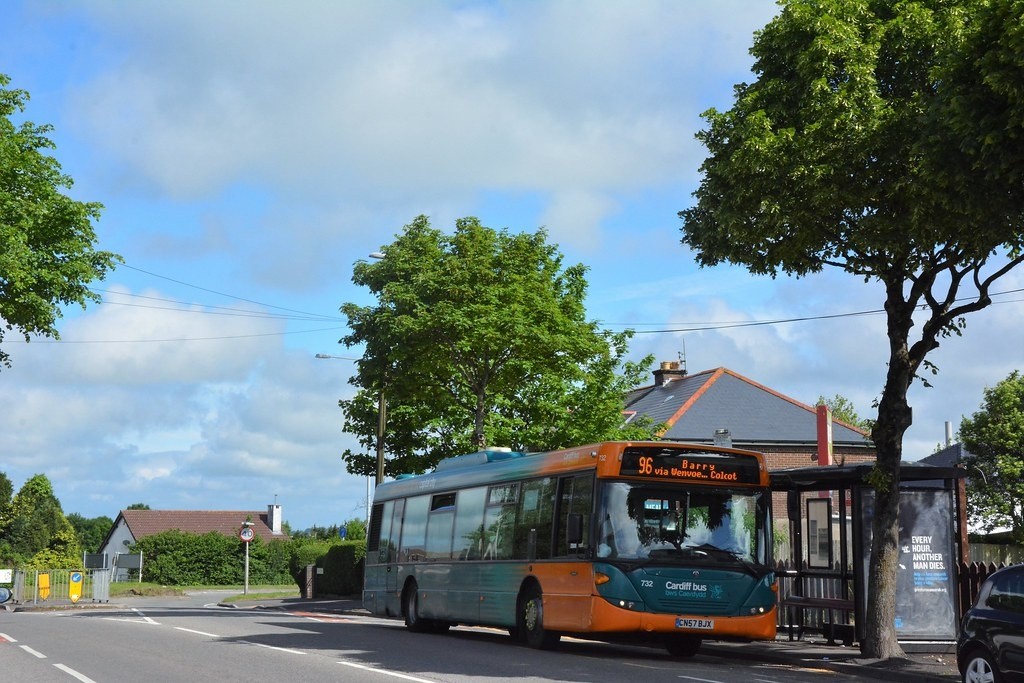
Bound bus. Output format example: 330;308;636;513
362;440;800;658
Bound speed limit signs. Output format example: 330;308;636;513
239;526;255;542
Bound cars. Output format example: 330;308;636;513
957;564;1024;683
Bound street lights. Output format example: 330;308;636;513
315;354;386;487
241;522;255;595
